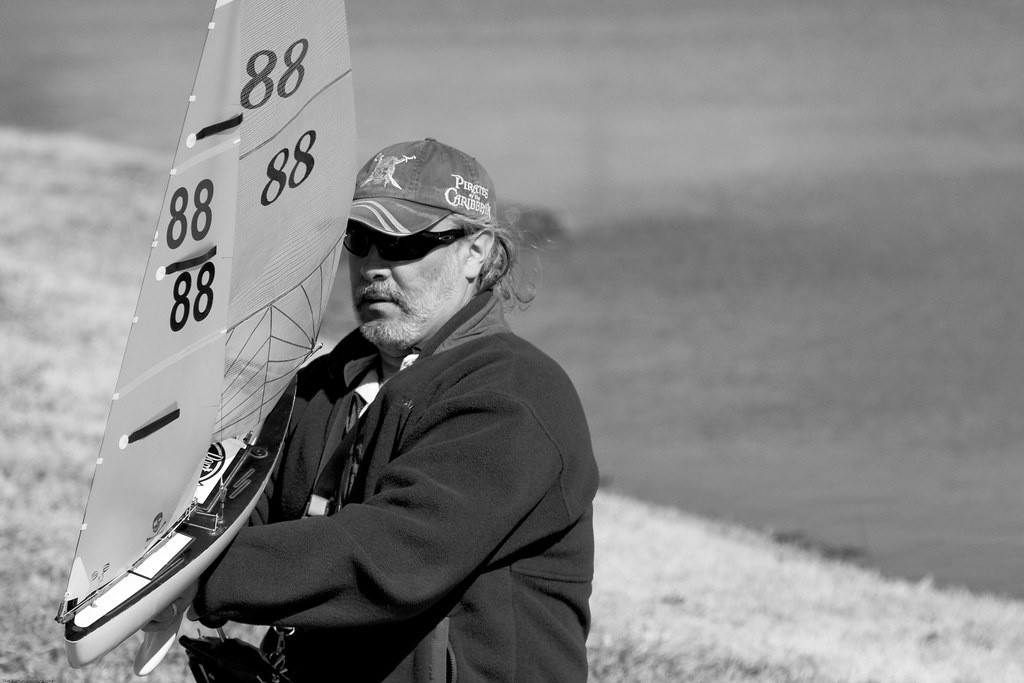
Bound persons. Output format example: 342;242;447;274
141;137;603;683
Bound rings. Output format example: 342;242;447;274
171;604;178;617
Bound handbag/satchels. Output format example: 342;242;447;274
179;636;290;682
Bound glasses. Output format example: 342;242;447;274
343;219;481;262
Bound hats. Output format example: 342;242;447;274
348;137;498;238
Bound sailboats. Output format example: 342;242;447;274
50;0;360;677
